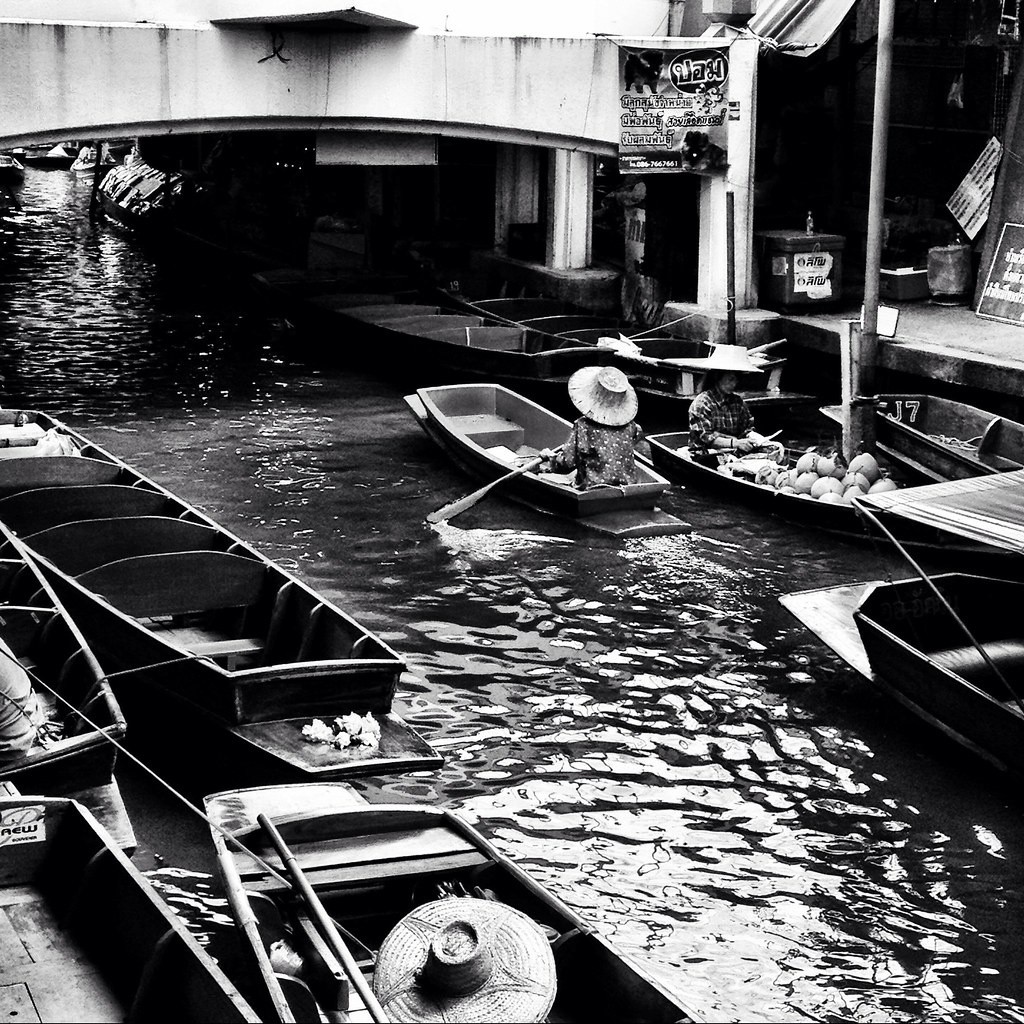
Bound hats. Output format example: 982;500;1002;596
657;344;764;374
372;899;557;1024
47;144;68;157
568;366;638;426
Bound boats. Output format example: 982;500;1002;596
0;409;444;789
0;796;264;1023
326;274;1024;786
0;514;138;879
1;142;207;237
199;783;703;1023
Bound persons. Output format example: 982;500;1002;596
541;366;640;491
0;638;35;761
688;345;785;468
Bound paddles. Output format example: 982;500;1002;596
746;338;787;356
256;812;391;1024
424;442;569;525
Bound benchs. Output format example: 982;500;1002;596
926;636;1024;689
184;639;264;672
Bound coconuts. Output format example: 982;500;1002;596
754;451;896;506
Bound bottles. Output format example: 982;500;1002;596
806;210;814;236
953;232;964;246
